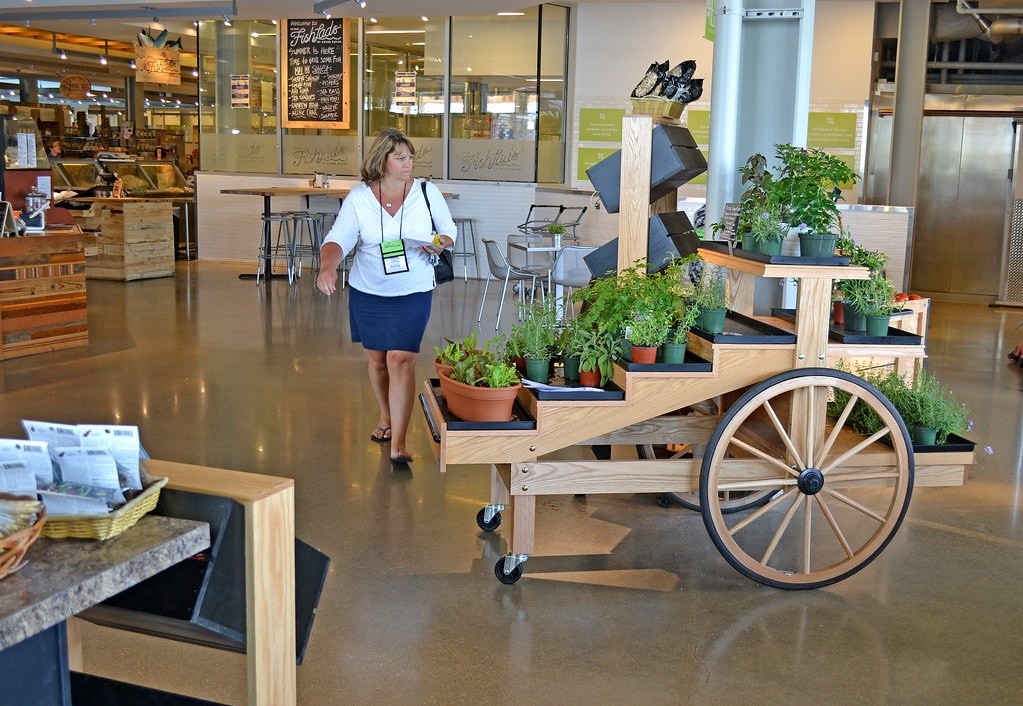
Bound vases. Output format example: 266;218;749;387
914;426;938;447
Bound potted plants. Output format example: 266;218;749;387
739;143;896;336
435;255;723;423
550;224;565;247
134;28;184;85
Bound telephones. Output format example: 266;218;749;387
112;179;122;197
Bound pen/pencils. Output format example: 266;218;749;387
438;247;444;248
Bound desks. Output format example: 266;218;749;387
0;515;209;706
0;223;88;361
219;186;351;280
509;242;587;309
60;195;194;280
326;193;452;286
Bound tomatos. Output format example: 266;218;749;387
892;293;921;302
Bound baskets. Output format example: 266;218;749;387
630;95;686;119
1;493;47;581
41;476;168;541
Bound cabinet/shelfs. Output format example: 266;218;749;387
1;114;199;225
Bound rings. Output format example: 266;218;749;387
438;250;441;253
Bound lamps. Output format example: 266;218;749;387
25;20;31;26
90;18;96;25
355;0;367;8
322;9;332;19
223;14;231;26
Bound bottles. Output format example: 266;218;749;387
24;186;47;225
39;121;158;157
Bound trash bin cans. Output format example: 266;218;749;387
173;206;181;260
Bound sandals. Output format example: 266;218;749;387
391;448;413;464
371;426;391;441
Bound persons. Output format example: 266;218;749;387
317;129;457;462
47;138;61;158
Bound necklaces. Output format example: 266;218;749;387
382;182;392;207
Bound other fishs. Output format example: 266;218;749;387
139;28;184;50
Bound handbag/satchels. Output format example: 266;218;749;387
422;181;454;284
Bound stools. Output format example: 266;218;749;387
255;210;355;289
449;218;480;283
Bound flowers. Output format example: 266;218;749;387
829;355;994;455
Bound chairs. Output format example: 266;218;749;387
478;234;599;328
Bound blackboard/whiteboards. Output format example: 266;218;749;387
280;17;350;130
720;203;742;241
0;202;17;233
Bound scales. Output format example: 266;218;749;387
97;151;130;159
